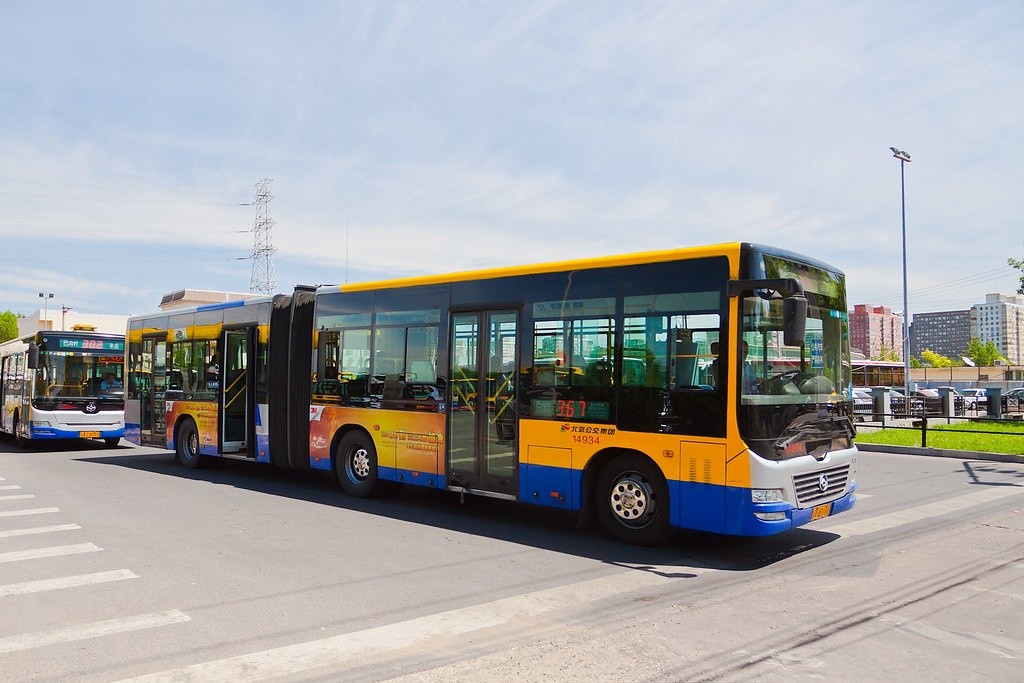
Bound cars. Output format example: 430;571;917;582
954;389;988;410
1001;388;1024;404
842;387;939;411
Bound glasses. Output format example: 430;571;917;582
744;350;748;354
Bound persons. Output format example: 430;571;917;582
741;340;768;395
101;373;122;390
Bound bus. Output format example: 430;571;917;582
125;242;858;543
0;330;125;447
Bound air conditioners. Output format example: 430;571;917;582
994;360;1005;366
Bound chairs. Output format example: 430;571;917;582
127;363;209;391
316;351;646;424
86;377;103;395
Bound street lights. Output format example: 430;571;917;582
890;147;911;414
39;293;54;330
